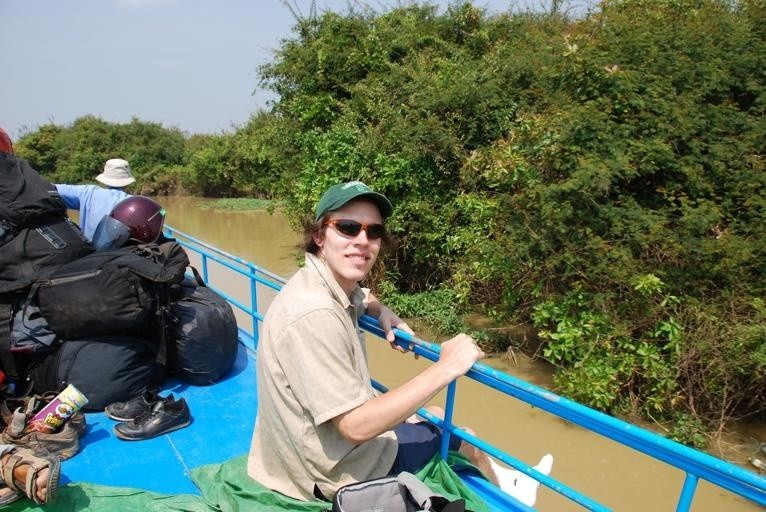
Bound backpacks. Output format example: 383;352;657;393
1;220;239;409
0;150;67;225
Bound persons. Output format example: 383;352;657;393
253;179;557;511
51;158;141;241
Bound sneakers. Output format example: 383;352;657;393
112;397;192;441
1;394;87;438
0;407;80;461
105;392;174;421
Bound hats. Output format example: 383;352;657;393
315;181;392;220
95;159;137;187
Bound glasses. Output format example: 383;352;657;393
325;219;386;241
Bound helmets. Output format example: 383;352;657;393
92;197;165;252
